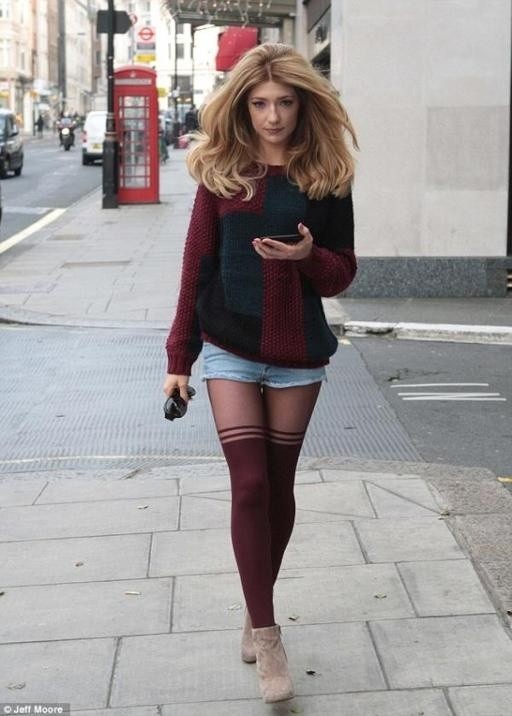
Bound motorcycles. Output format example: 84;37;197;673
56;118;77;151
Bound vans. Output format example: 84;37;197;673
81;110;125;166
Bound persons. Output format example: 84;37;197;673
183;104;199;135
163;41;359;704
34;116;45;138
57;112;77;147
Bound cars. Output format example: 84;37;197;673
154;104;200;147
0;108;25;180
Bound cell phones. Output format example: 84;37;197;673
261;233;305;242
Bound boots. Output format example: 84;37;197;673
239;597;296;705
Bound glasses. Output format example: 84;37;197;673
163;384;197;422
